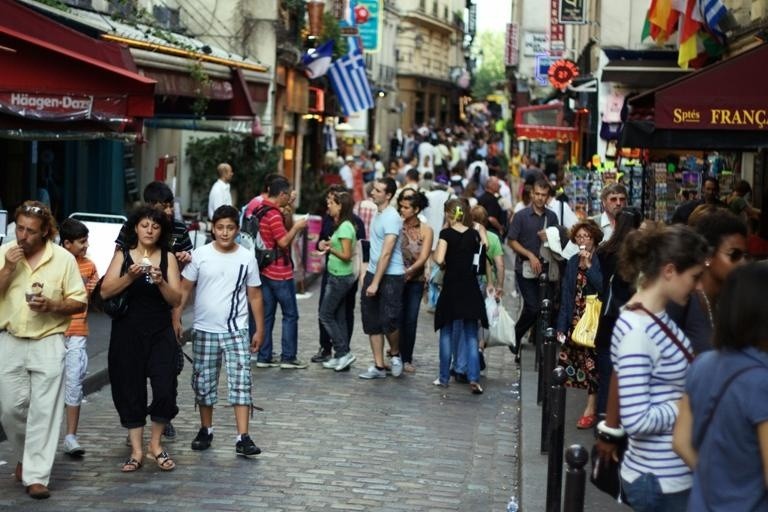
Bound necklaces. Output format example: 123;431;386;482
695;283;716;332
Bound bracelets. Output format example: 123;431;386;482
593;421;625;444
408;267;416;275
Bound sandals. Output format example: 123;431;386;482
404;361;416;372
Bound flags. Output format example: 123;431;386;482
328;21;374;116
640;0;727;71
303;41;334;79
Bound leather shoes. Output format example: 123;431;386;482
454;373;469;385
25;484;50;500
576;414;598;429
162;422;178;441
15;460;23;482
509;339;521;355
63;434;86;457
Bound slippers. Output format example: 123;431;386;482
145;449;176;471
120;455;142;471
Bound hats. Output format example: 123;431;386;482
416;126;430;137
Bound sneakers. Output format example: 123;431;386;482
478;346;486;372
390;352;404;377
322;359;349;370
255;358;280;368
279;358;308;369
334;352;357;372
191;426;214;451
357;366;387;379
311;351;331;361
235;433;261;456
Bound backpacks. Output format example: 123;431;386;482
241;205;287;269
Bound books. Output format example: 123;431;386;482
300;212;310;237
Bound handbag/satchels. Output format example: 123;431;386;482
570;289;603;350
523;216;548;279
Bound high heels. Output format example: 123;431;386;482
432;377;449;389
469;380;484;395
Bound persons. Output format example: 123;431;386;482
253;178;308;369
420;172;447;191
741;217;767;258
542;184;578;232
100;206;182;473
352;181;378;287
417;182;459;315
543;159;568;200
608;218;707;512
113;181;194;448
311;184;371;363
728;178;761;226
516;158;553;203
55;218;99;456
509;148;522;207
386;188;434;374
449;176;464;194
448;169;470;195
506;179;562;354
171;205;265;459
358;176;408;380
560;182;629;260
594;202;752;472
468;166;488;201
430;197;490;394
107;200;142;223
35;170;51;212
448;205;495;377
519;154;532;177
477;176;506;303
392;169;428;218
204;163;240;245
672;177;731;224
672;259;767;512
0;200;89;500
386;116;506;175
586;208;654;430
394;189;437;305
319;192;357;371
553;219;619;429
489;171;513;244
324;142;383;185
241;173;297;361
509;184;536;224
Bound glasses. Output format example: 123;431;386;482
716;247;751;263
19;206;48;216
574;233;591;240
